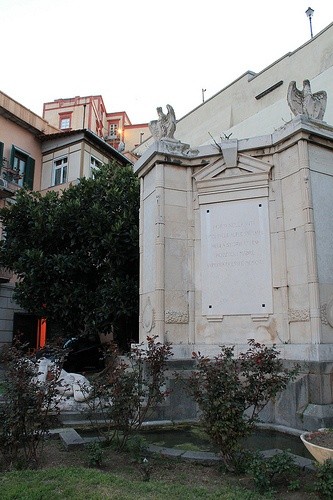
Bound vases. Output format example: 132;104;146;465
300;428;333;465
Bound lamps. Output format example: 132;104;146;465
305;7;317;39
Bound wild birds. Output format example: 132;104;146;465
287;79;328;120
148;104;177;140
